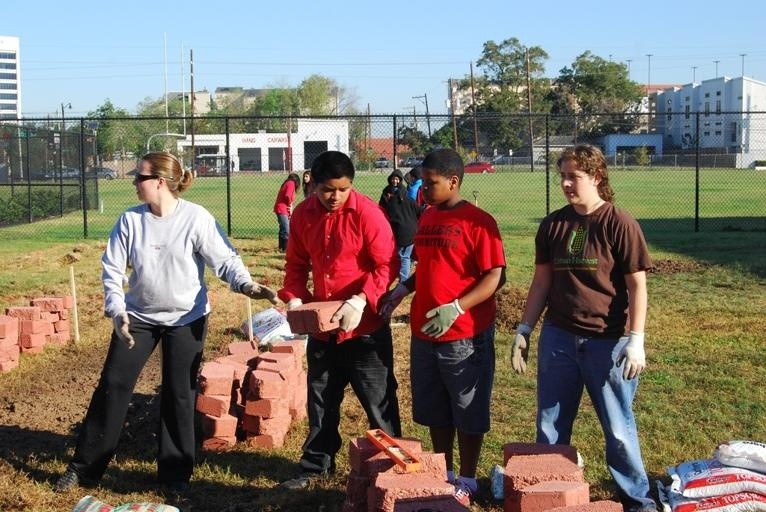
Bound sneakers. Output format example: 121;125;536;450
54;466;81;492
445;471;455;486
277;471;328;492
457;474;483;500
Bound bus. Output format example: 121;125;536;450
195;152;235;179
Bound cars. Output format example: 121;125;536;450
464;161;495;174
84;167;119;181
374;156;389;168
399;156;425;167
51;168;79;179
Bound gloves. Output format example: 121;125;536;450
108;307;136;351
419;299;465;339
286;298;303;310
616;329;646;379
242;282;278;305
511;324;535;376
376;283;409;321
330;295;368;344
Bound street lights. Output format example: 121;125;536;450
59;103;74;170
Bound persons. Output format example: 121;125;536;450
512;142;658;512
273;165;425;283
51;150;278;498
380;149;506;500
273;151;403;476
